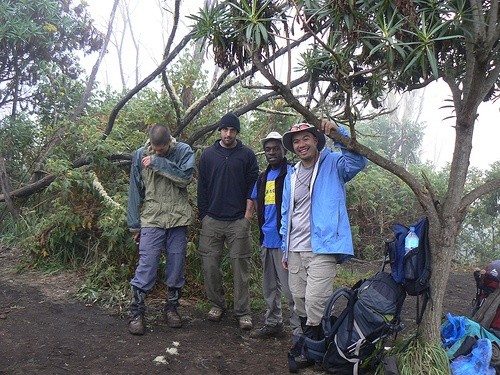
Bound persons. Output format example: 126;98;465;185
250;131;303;339
196;113;259;328
127;122;196;335
280;117;367;371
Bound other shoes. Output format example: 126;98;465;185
128;316;146;334
239;316;253;330
166;311;181;328
208;307;223;321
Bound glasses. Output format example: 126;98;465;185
289;124;310;131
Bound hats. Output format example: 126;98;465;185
261;132;283;146
282;122;326;154
218;112;240;132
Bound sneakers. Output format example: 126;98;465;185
250;324;284;338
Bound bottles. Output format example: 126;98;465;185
405;227;419;279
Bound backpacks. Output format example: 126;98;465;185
385;217;431;296
286;273;406;375
